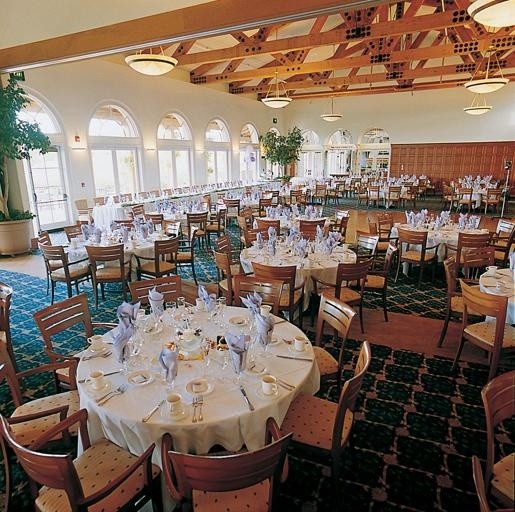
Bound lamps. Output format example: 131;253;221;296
320;96;343;123
464;44;511;94
124;43;179;77
260;51;293;110
467;0;515;28
463;92;493;115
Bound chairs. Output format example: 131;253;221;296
38;180;240;310
437;257;515;383
472;370;515;511
378;212;515;286
213;209;398;333
357;177;510;215
239;177;361;217
1;273;370;511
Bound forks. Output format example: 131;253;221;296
191;395;206;422
96;384;127;406
82;351;112;360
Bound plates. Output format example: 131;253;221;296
287;345;315;357
86;380;111;393
85;343;111;354
162;406;189;421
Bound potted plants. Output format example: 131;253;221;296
0;70;56;258
257;124;309;188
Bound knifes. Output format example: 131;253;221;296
143;399;164;423
78;370;118;384
276;355;312;362
239;385;254;410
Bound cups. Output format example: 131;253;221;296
88;336;103;351
166;393;182;415
294;336;310;351
85;371;104;390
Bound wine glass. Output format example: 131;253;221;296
71;193;206;248
253;203;353;265
406;210;515;292
115;297;283;400
